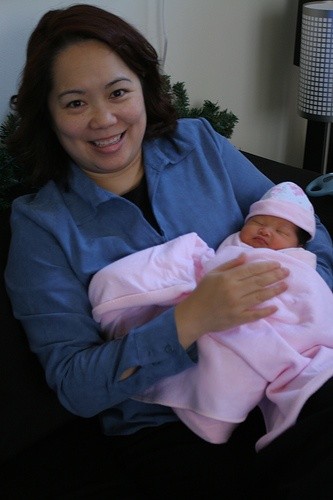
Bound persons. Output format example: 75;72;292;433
1;4;333;499
88;180;333;450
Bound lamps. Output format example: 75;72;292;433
295;0;333;176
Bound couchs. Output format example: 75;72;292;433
0;148;333;500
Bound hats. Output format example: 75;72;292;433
243;182;315;243
